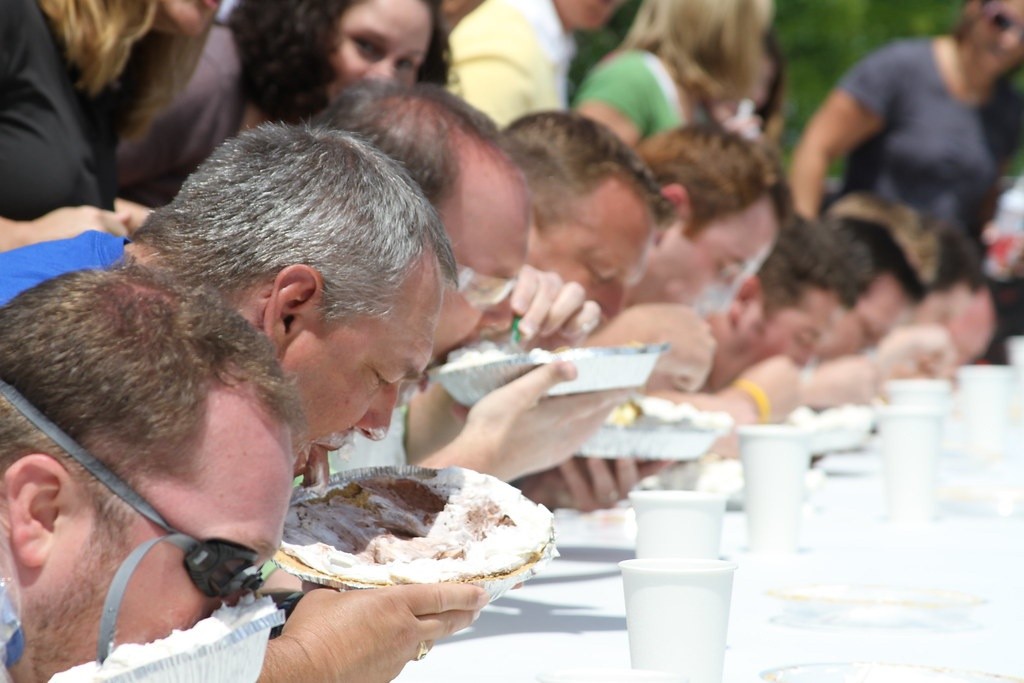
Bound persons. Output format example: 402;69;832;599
1;0;1024;683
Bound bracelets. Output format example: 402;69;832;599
732;379;770;425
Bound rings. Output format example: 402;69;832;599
609;491;618;501
415;641;428;661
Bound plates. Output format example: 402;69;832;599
569;411;730;463
432;331;668;407
266;467;559;615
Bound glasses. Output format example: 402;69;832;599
183;537;305;641
984;12;1023;30
454;262;520;310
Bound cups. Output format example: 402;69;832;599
616;553;730;680
876;337;1022;522
733;424;808;529
627;488;728;553
